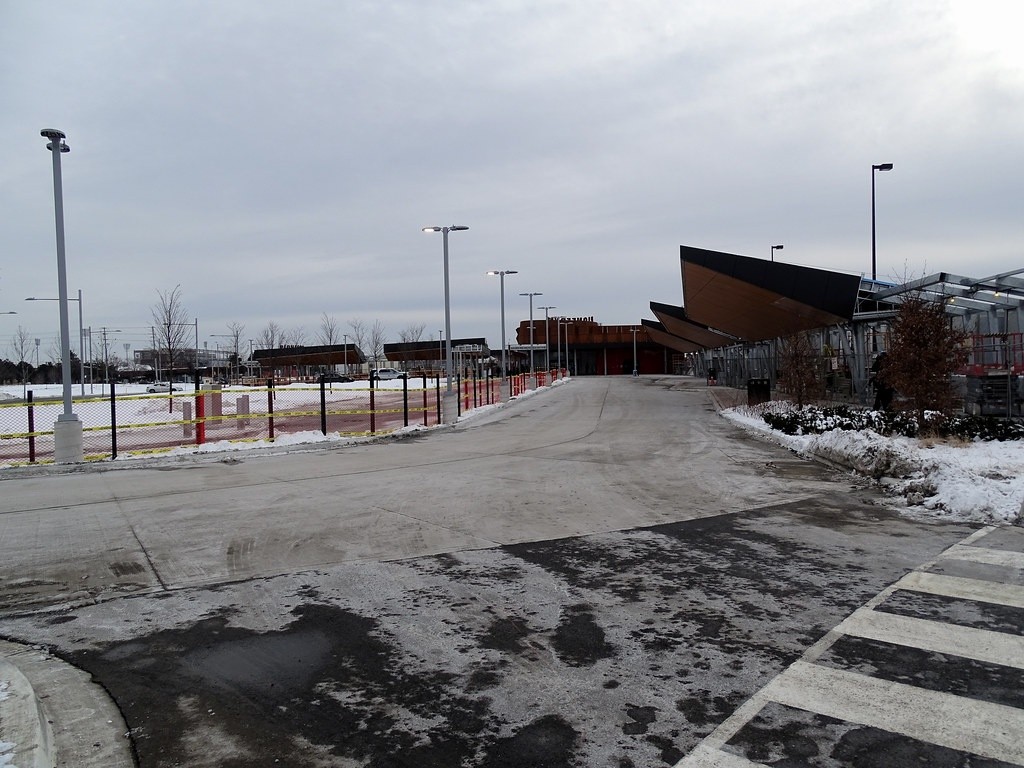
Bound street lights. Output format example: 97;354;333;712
771;245;783;262
519;293;544;390
437;330;444;372
24;289;86;396
871;163;894;280
88;325;122;394
163;318;199;371
421;224;470;428
537;306;557;387
343;334;348;375
487;270;519;403
559;322;574;378
629;329;641;377
40;126;78;419
551;315;567;380
210;331;240;386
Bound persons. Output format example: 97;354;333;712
871;352;894;413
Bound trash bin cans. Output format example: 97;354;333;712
707;368;718;380
746;378;771;406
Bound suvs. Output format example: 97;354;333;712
367;367;411;381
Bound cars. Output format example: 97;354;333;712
145;383;184;394
316;372;355;383
